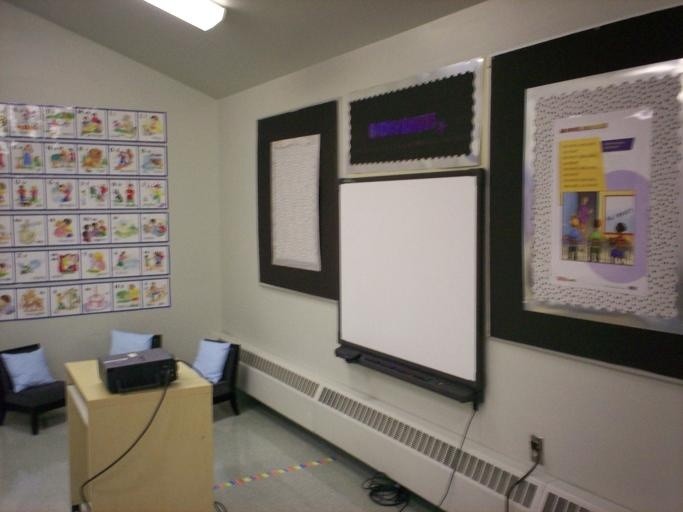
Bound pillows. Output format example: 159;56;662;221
0;347;59;393
110;328;154;355
192;340;231;384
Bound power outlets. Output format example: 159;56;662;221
530;433;545;466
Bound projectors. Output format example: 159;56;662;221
99;347;176;394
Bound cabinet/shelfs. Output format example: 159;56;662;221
62;360;215;512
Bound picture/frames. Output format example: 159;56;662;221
482;0;683;385
255;98;339;301
337;57;482;175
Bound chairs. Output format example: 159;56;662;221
188;339;242;419
0;343;67;436
151;334;163;347
